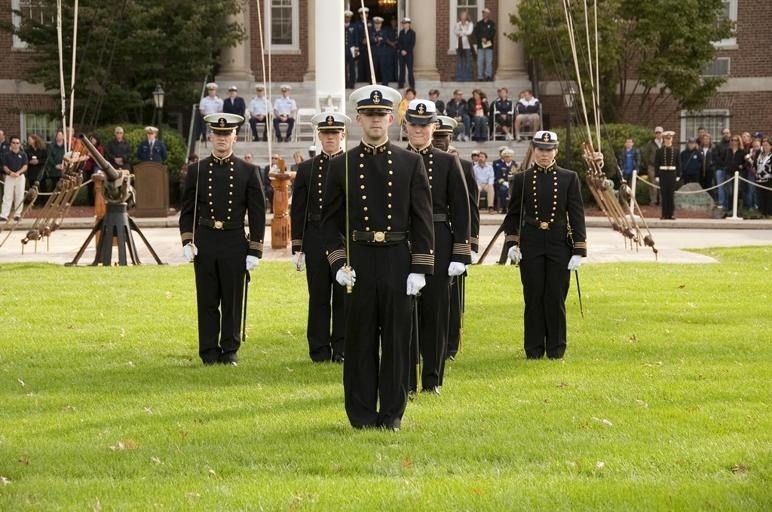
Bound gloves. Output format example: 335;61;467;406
183;241;198;262
335;266;356;287
292;252;306;266
406;273;426;295
471;250;477;263
507;245;522;261
568;254;587;271
448;262;465;276
245;255;259;271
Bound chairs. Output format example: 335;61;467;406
397;97;544;143
237;107;319;144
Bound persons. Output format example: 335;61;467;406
405;98;471;400
472;146;518;214
472;9;496;81
222;85;246;141
244;84;273;142
505;131;587;359
419;117;480;360
398;88;540;142
245;153;262;179
344;7;416;89
290;111;353;364
64;126;104;207
1;130;65;221
264;153;287;213
188;155;198;165
620;126;771;220
107;126;130;170
454;12;474;81
320;84;435;429
136;126;168;165
198;83;224;142
273;85;297;142
179;112;266;366
291;152;304;171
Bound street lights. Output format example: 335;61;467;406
152;83;165;141
561;80;575;170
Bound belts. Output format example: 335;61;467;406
524;215;566;230
307;212;320;221
659;165;676;170
433;213;447;223
197;216;245;231
348;229;407;243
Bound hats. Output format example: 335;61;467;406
255;84;264;91
144;126;158;135
205;83;219;90
654;126;676;138
228;85;237;91
203;112;245;134
311;111;352;132
348;84;402;114
280;84;291;92
432;116;458;136
531;131;559;150
403;99;437;125
